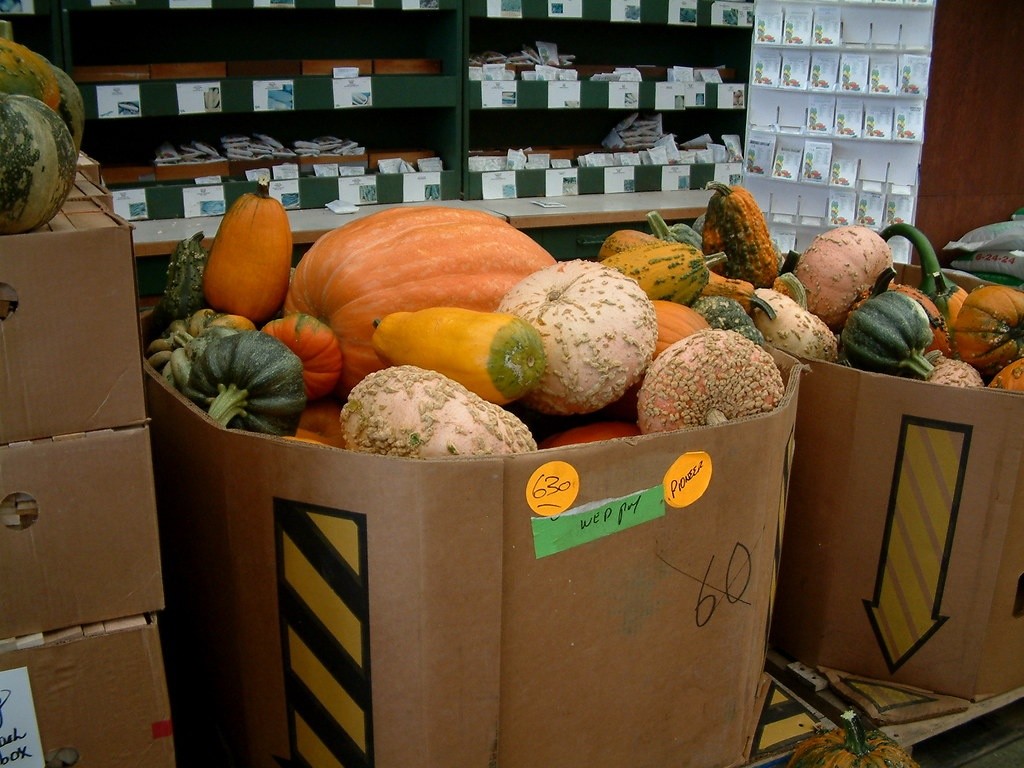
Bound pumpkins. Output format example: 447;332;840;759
0;18;86;234
784;709;923;768
141;173;1024;458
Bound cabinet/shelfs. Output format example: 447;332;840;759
0;0;755;222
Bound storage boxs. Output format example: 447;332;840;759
0;152;1024;768
